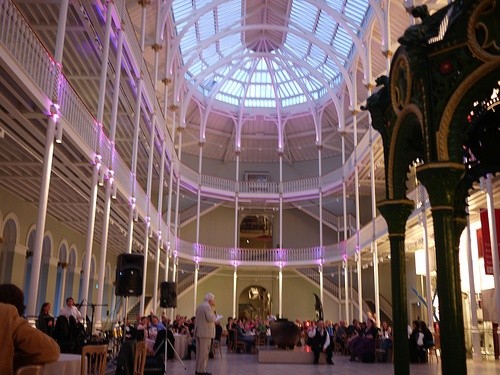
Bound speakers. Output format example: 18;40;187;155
160;281;178;308
115;253;145;297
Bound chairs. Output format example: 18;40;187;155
0;313;440;374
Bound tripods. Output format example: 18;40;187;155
153;308;187;373
106;296;127;367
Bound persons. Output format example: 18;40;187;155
325;316;439;364
221;311;318;351
0;284;193;375
305;321;334;366
193;292;224;375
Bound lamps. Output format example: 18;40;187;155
94;157;179;264
56;118;63;144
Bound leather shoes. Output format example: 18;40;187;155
195;371;212;375
